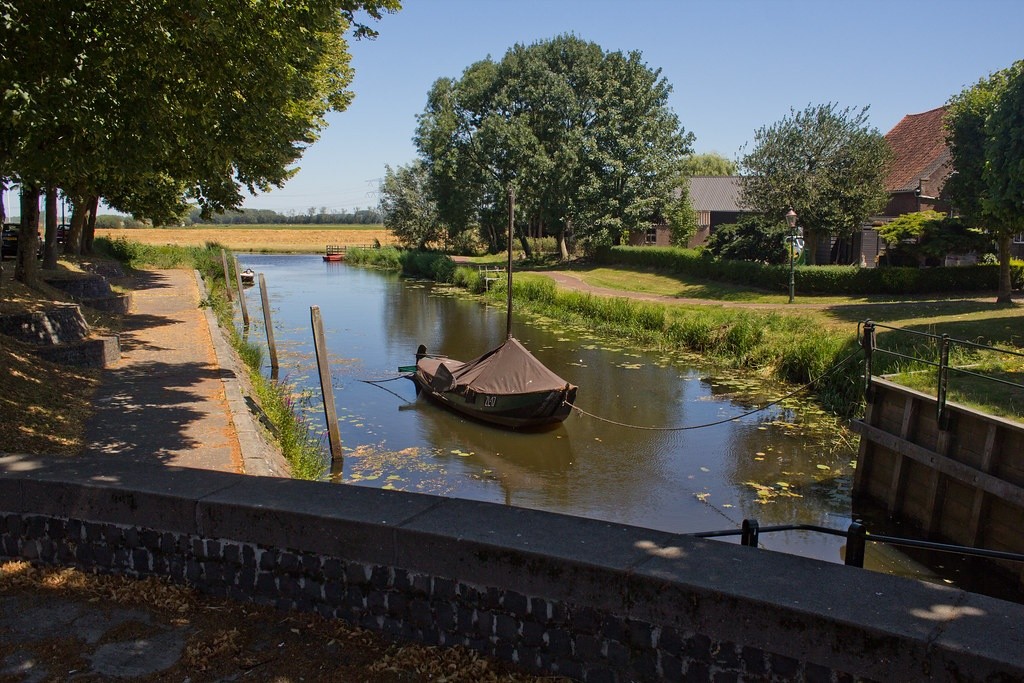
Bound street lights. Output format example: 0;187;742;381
783;206;798;305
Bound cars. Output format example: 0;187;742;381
57;224;72;244
1;223;42;262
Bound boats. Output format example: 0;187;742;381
399;187;579;432
